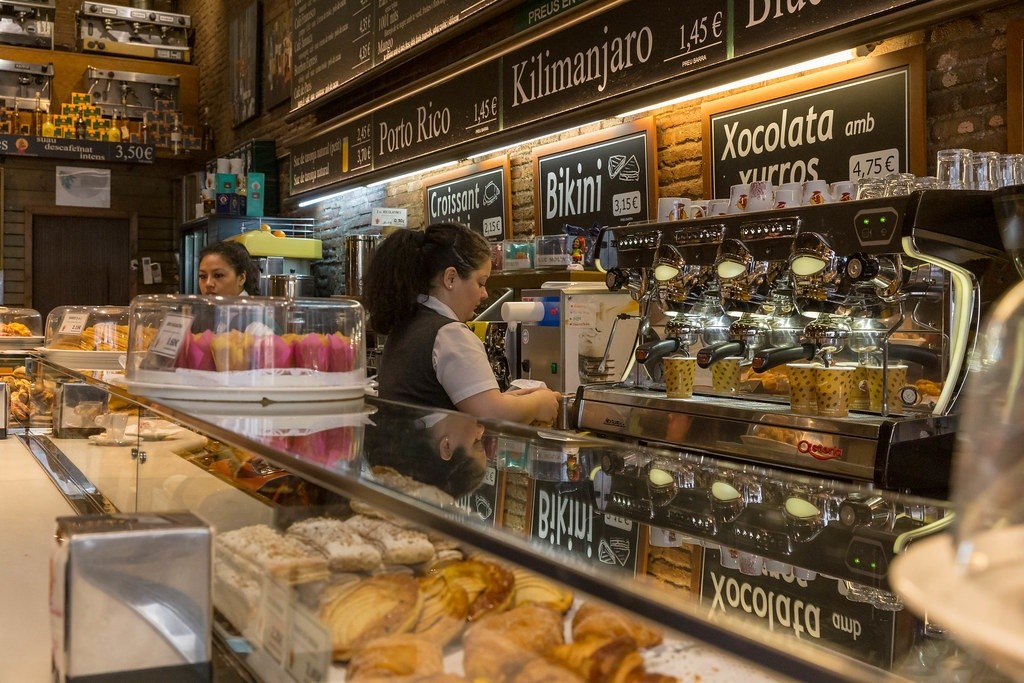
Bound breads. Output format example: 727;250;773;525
217;465;679;683
888;333;919;339
0;323;159;420
747;370;788;390
915;379;945;397
755;423;833;453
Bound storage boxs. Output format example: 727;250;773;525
215;172;265;220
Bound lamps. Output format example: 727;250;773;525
291;40;883;208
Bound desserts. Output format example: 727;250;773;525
259;426;356;465
176;328;355;372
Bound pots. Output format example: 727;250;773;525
266;268;324;299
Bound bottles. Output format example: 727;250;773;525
11;91;214;153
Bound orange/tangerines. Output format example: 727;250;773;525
273;230;285;237
261;224;271;233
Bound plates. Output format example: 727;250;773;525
125;425;186;441
89;435;144;446
67;418;106;430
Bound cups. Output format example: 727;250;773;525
94;413;129;440
578;329;612;385
835;362;908;414
195;157;244;218
661;356;698;399
74;401;103;426
648;525;951;640
787;364;856;419
657;149;1024;223
710;356;744;397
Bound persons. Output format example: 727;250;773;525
568;238;586;262
365;401;487;498
368;223;561;425
563;455;582;481
193;240;282;333
